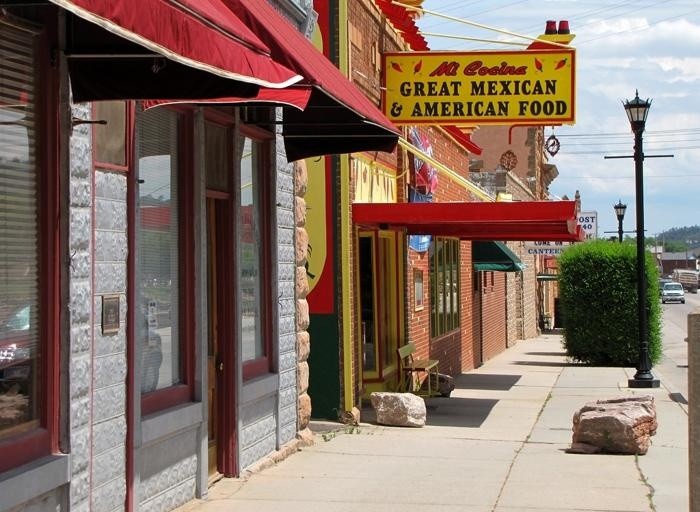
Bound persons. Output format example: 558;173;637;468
104;304;119;325
138;329;163;395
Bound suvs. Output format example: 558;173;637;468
654;266;699;305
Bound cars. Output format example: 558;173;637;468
0;280;169;415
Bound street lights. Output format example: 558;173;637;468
604;198;648;246
603;87;675;389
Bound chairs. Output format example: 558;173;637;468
397;341;442;397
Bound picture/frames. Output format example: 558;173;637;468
413;267;424;312
